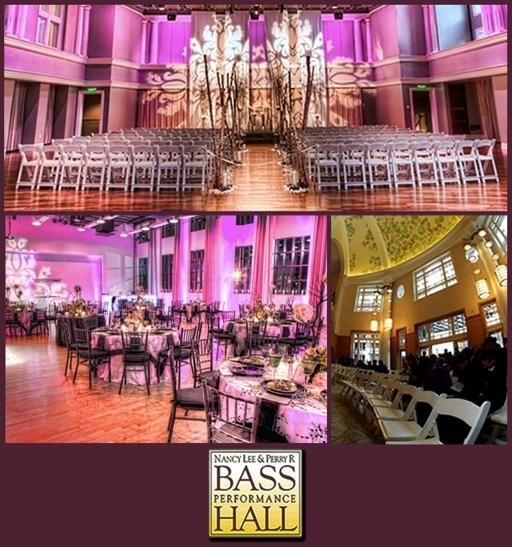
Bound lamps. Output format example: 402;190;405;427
463;242;490;300
371;287;380;333
5;217;14;239
477;229;507;287
334;8;344;19
165;11;179;19
249;8;259;19
383;286;393;329
31;215;179;238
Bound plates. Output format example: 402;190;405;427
229;355;328;404
93;329;172;335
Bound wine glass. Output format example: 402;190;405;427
261;348;283;380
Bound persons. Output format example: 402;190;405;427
338;336;507;444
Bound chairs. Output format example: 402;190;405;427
332;361;507;445
